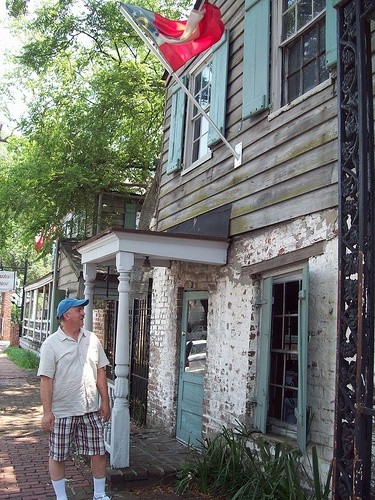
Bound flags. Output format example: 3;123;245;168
120;0;225;73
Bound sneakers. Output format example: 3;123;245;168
92;494;112;500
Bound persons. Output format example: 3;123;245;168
37;298;110;500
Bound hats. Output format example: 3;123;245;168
56;295;90;319
